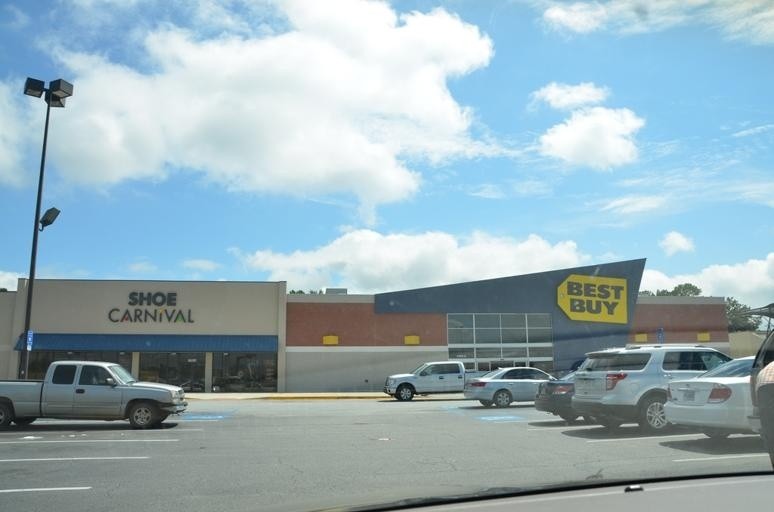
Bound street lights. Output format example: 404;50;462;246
18;75;73;381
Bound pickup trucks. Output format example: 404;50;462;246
383;361;491;401
0;360;188;430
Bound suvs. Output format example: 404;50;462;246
570;343;733;434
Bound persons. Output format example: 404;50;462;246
755;361;774;472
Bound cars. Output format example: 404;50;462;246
663;356;761;438
463;367;600;425
